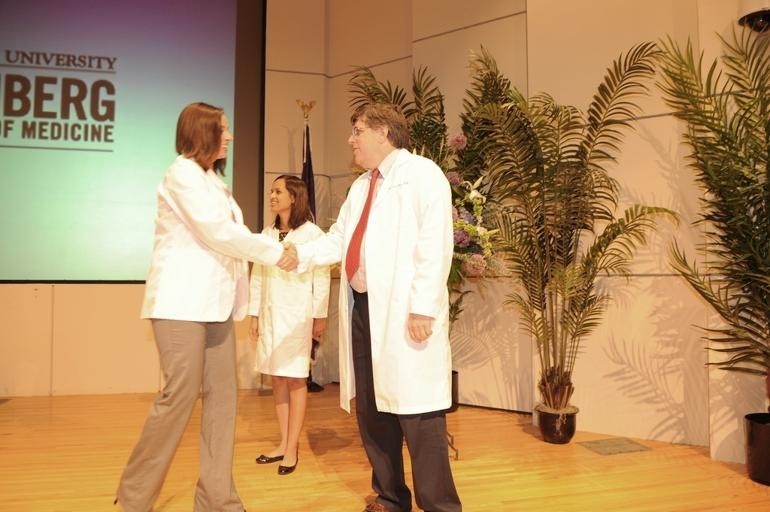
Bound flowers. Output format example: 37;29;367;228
437;134;499;280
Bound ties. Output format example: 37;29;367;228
344;167;380;284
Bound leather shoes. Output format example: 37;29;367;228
256;452;283;464
279;454;299;475
364;501;391;512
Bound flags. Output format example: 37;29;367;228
301;123;317;225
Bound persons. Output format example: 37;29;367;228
114;100;300;512
283;100;463;512
246;173;333;475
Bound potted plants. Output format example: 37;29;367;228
471;41;680;445
654;16;770;486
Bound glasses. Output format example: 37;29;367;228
352;126;373;138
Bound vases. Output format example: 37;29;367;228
447;370;458;413
